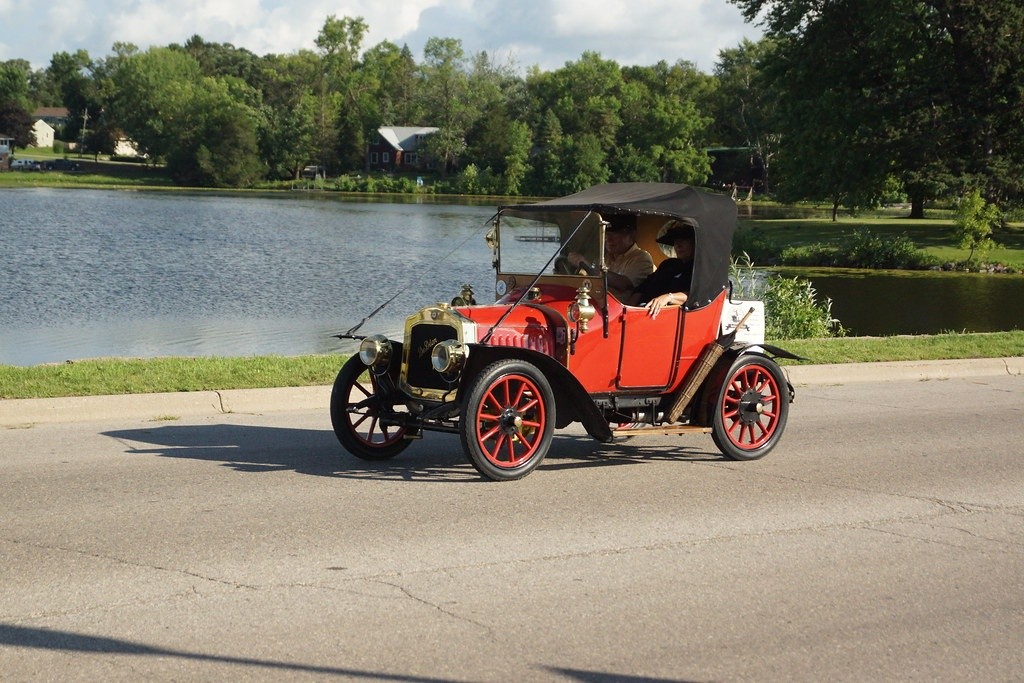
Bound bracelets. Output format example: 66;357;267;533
669;293;674;304
590;263;595;270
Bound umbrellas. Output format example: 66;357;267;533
666;307;755;423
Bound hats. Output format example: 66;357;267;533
603;214;637;230
656;221;695;246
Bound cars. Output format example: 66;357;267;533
330;182;809;482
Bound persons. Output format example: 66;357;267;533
629;225;695;319
567;217;652;304
